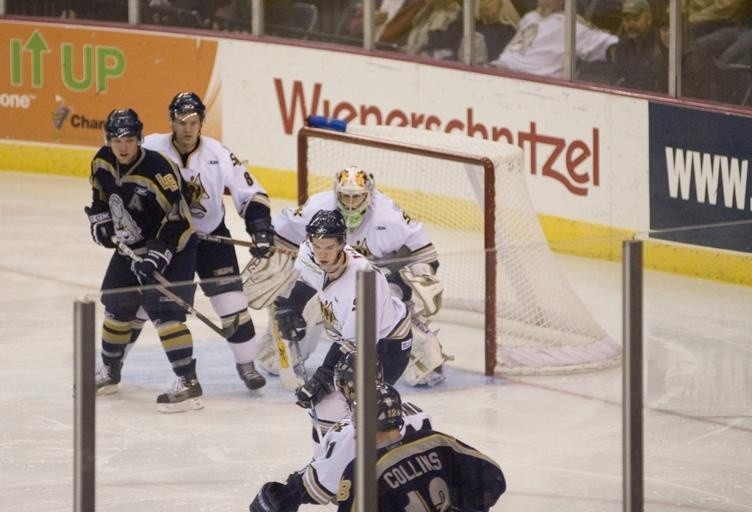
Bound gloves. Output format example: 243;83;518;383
137;248;173;290
294;367;335;408
249;482;299;512
88;207;117;247
248;220;279;258
276;298;308;340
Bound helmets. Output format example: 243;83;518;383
305;208;346;248
332;167;374;227
105;109;144;145
352;383;402;433
333;350;384;401
169;92;206;127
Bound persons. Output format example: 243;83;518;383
337;382;506;512
122;91;276;390
249;350;433;512
270;165;446;387
1;0;751;106
273;210;413;484
84;109;203;404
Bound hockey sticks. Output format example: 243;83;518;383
111;234;239;338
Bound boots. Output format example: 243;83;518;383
236;361;265;390
156;370;202;404
93;361;123;388
